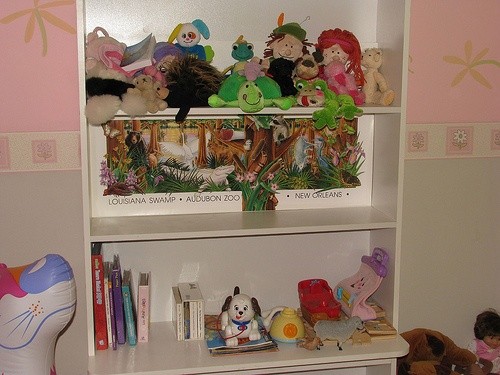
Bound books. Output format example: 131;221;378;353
92;243;152;350
171;281;205;342
119;32;157;73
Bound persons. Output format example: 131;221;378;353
466;310;500;375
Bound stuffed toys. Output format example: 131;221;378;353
398;310;479;375
84;13;395;130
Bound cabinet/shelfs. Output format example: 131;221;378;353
74;0;410;375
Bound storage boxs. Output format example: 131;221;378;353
298;278;340;327
